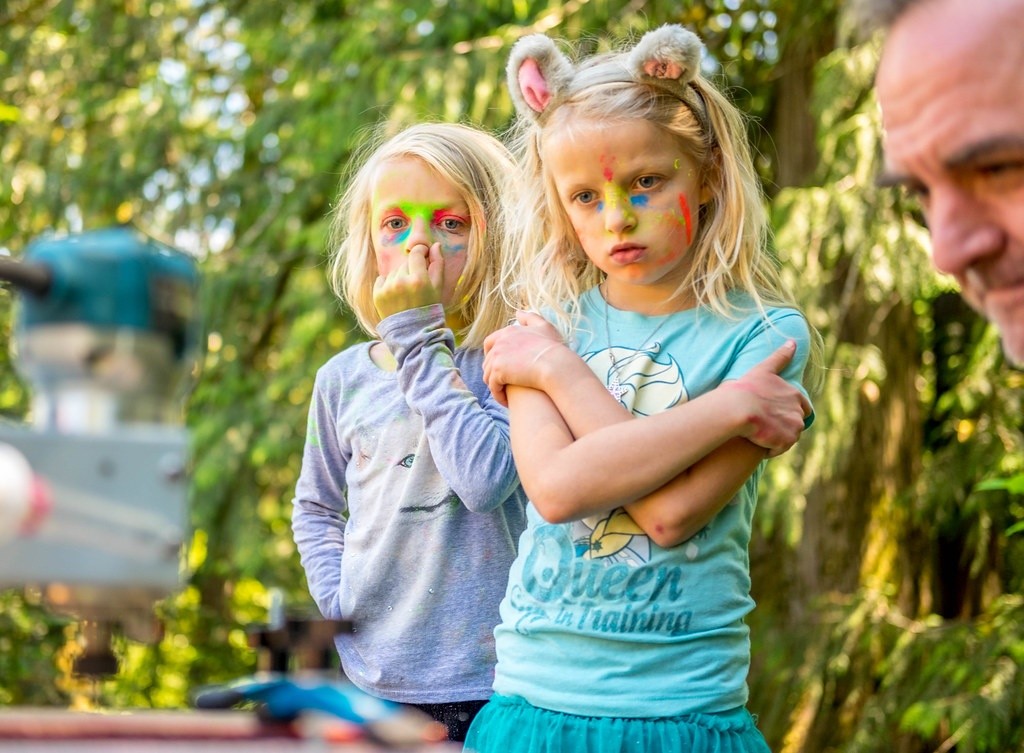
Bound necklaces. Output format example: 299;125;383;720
598;282;697;403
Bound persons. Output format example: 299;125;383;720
286;120;527;743
462;25;818;753
858;0;1023;365
